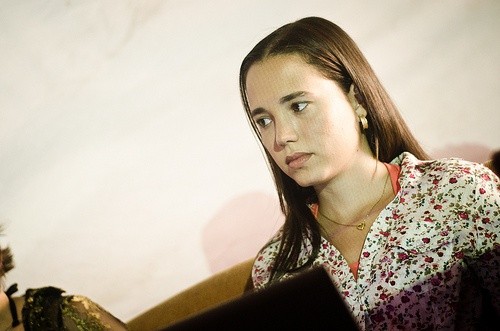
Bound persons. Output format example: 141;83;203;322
239;16;500;331
0;224;130;331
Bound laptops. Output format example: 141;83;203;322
160;266;362;331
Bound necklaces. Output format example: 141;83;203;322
320;174;390;230
